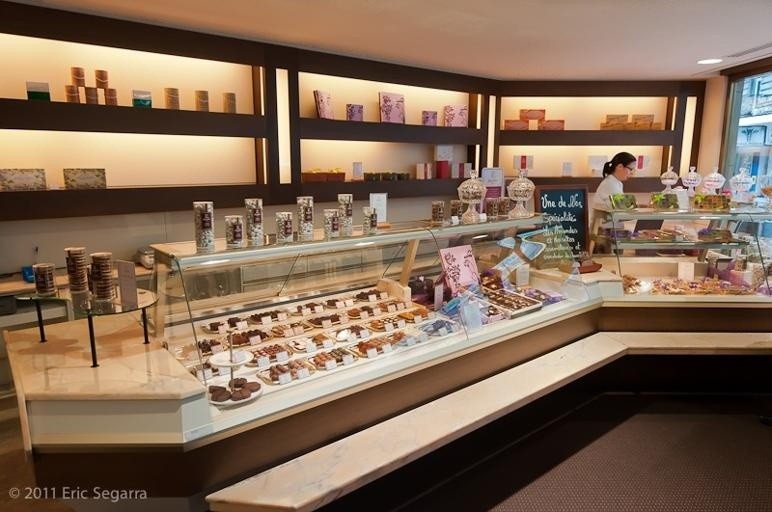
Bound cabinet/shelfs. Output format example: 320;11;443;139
150;213;604;434
0;0;292;223
489;78;707;194
587;206;772;301
288;46;489;205
0;262;156;421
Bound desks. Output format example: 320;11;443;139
14;288;159;368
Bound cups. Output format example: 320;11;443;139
88;252;117;303
23;266;35;283
364;172;411;181
64;247;88;293
66;66;237;115
33;262;58;296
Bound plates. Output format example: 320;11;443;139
173;289;460;406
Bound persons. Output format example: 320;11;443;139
587;153;637;245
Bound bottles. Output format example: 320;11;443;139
296;196;314;242
244;198;265;248
274;211;294;244
337;194;353;237
224;215;244;249
193;201;215;254
362;206;379;237
322;208;340;238
706;252;772;291
431;197;510;227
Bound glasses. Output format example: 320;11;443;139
626;166;634;171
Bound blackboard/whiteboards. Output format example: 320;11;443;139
534;184;590;269
